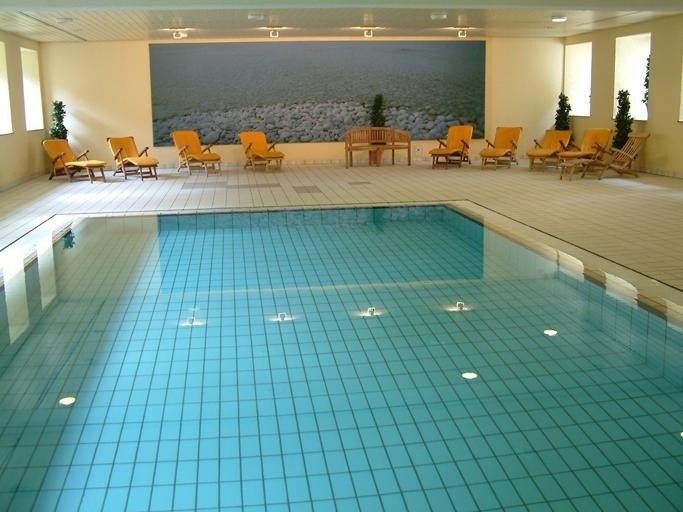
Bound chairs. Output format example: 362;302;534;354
525;127;574;175
555;131;653;184
170;129;222;178
107;135;160;181
41;138;107;184
555;126;614;178
478;126;523;171
237;131;286;171
425;124;473;170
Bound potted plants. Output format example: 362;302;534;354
606;89;637;173
364;91;393;169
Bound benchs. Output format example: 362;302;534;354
344;127;413;169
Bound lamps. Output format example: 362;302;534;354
458;31;466;37
364;30;373;38
269;31;279;37
456;300;465;311
188;317;195;325
367;306;375;316
279;313;286;321
552;16;567;23
173;31;182;39
